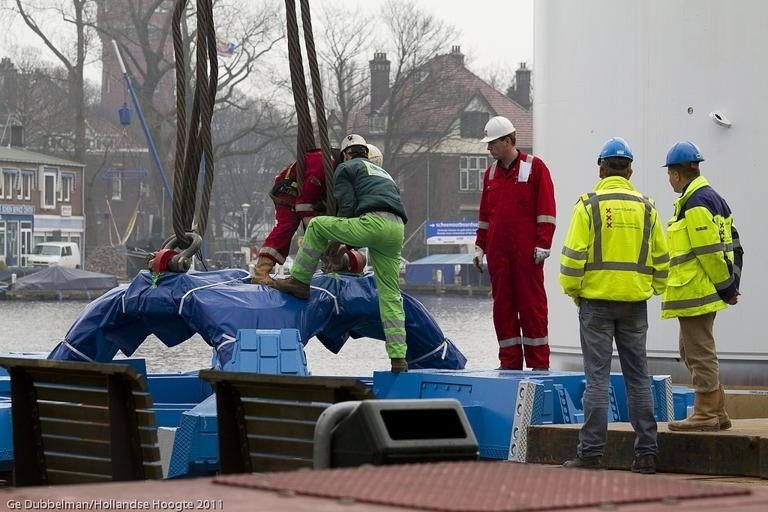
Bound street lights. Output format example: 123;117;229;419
240;202;250;241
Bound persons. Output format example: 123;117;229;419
558;137;670;473
472;116;557;372
273;133;408;372
660;142;744;432
250;144;383;284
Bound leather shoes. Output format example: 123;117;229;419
632;455;656;474
563;457;604;468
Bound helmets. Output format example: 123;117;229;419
598;137;633;165
340;134;383;167
480;116;515;143
661;141;704;167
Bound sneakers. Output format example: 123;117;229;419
391;359;408;372
273;276;310;299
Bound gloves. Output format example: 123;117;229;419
534;246;550;265
473;245;484;272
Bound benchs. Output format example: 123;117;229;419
199;367;375;477
1;358;162;484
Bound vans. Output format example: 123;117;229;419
27;240;82;271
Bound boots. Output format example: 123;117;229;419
668;384;732;431
252;256;279;289
